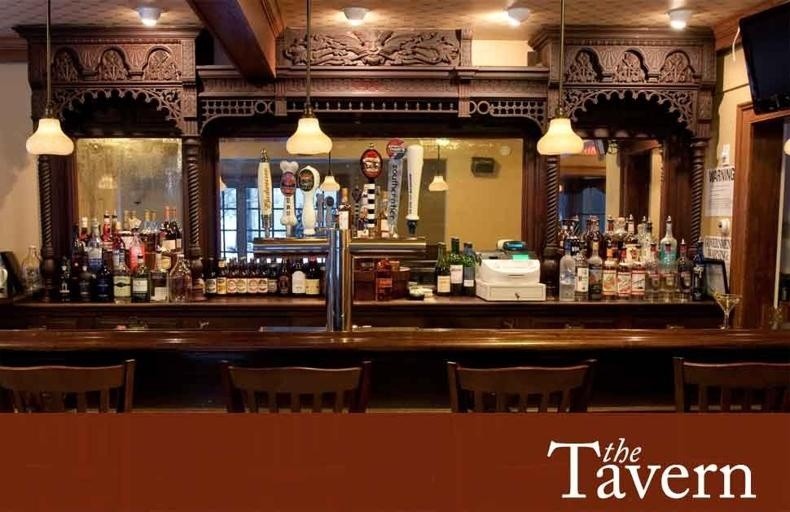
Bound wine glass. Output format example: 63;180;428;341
712;291;742;330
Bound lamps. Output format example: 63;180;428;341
505;0;533;24
320;152;341;192
130;6;169;27
282;0;334;157
24;0;75;157
339;6;372;24
426;142;449;194
664;1;700;32
534;1;586;159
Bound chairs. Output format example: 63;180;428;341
219;357;371;414
672;355;790;414
445;358;597;414
0;355;136;417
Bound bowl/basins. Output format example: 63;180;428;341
354;267;411;299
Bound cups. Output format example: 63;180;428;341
374;270;393;302
407;280;434;299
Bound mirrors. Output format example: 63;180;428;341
212;130;539;275
552;125;669;257
58;125;189;268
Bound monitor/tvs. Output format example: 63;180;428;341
738;2;790;116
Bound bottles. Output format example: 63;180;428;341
21;204;321;300
538;214;709;300
435;238;477;296
